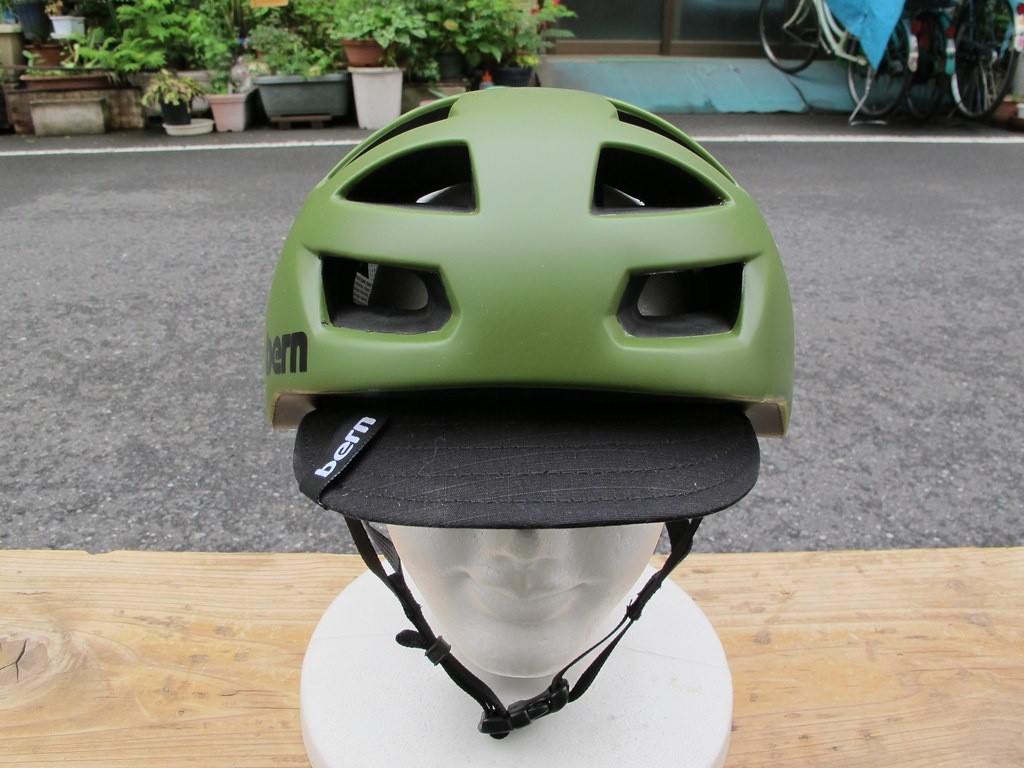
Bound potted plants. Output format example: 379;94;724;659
0;0;578;136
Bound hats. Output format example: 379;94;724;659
292;384;760;532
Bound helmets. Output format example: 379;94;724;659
272;102;795;524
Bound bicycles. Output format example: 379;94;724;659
757;0;1024;130
